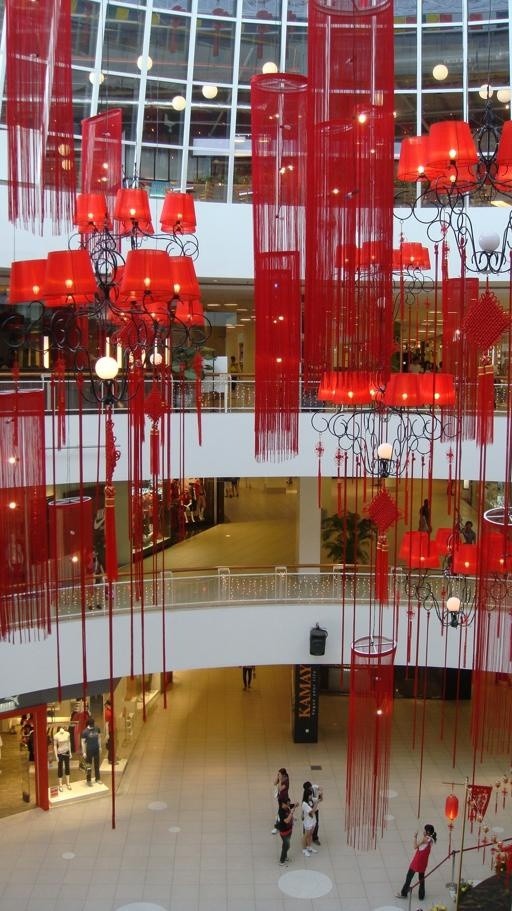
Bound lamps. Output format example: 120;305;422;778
9;248;212;406
392;1;512;277
68;1;201;279
397;528;512;627
335;240;434;319
310;369;462;479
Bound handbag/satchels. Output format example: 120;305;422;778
78;756;93;777
273;819;291;832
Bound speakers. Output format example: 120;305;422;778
310;630;326;656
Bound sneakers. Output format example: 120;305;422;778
395;892;407;898
302;838;321;858
271;827;280;834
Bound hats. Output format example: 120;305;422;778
87;719;95;728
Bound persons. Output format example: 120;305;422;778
408;356;443;374
239;666;256;691
81;719;103;786
19;696;113;764
393;823;437;901
418;498;429;532
275;797;299;866
182;478;206;523
54;727;73;792
301;789;321;857
228;356;242;392
270;767;289;834
302;781;324;845
460;521;476;545
